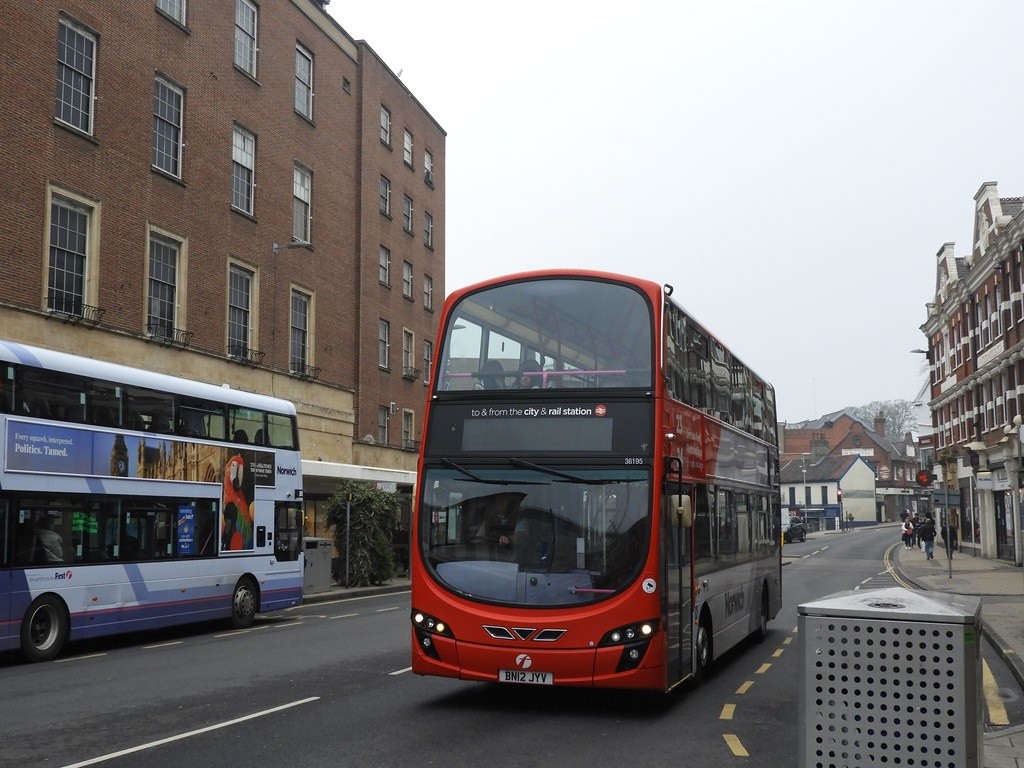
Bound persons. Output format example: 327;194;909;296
222;501;243;551
108;527;136;559
233;427;270;446
27;514;64;563
922;517;936;561
471;493;529;563
899;509;937;561
941;520;957;560
473;358;555;389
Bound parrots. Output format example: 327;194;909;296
221;455;253;551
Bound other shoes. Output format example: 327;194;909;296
905;547;908;549
908;547;911;549
927;557;930;560
930;553;933;559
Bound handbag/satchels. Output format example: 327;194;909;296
901;533;906;541
905;529;912;535
920;541;925;553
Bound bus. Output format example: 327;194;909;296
408;268;782;690
0;339;308;663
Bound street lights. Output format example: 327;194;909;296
938;455;958;577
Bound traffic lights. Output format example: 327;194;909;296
837;490;841;503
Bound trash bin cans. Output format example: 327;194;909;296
302;537;333;594
795;586;984;768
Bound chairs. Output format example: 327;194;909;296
481;360;543;389
22;396;273;447
35;538;215;567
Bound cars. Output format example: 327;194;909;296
781;516;807;543
